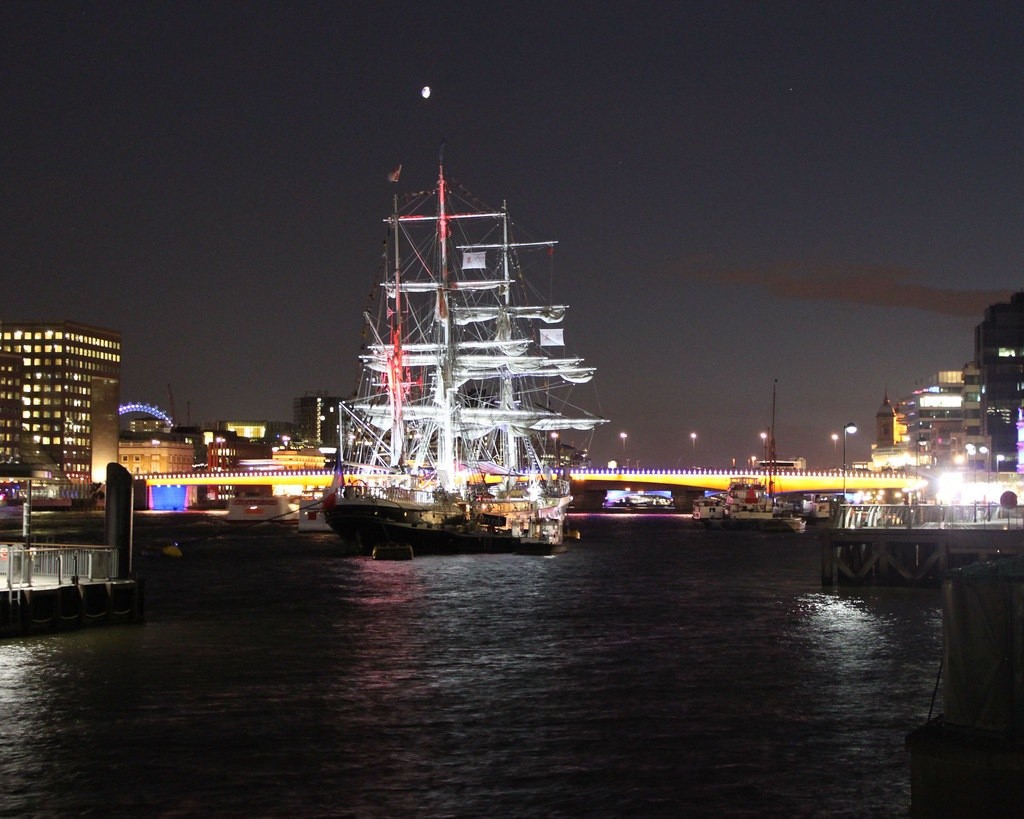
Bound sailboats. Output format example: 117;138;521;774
322;163;611;553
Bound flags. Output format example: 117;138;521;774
322;447;345;509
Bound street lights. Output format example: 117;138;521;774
691;433;696;449
916;437;927;505
979;447;990;504
620;433;627;450
761;433;767;467
834;435;837;448
965;449;976;505
842;422;857;528
996;455;1005;482
965;442;976;505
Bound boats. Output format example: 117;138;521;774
0;498;25;520
758;512;807;532
221;497;299;525
722;477;773;519
691;498;724;526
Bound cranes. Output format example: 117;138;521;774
187;401;192;427
168;383;176;425
